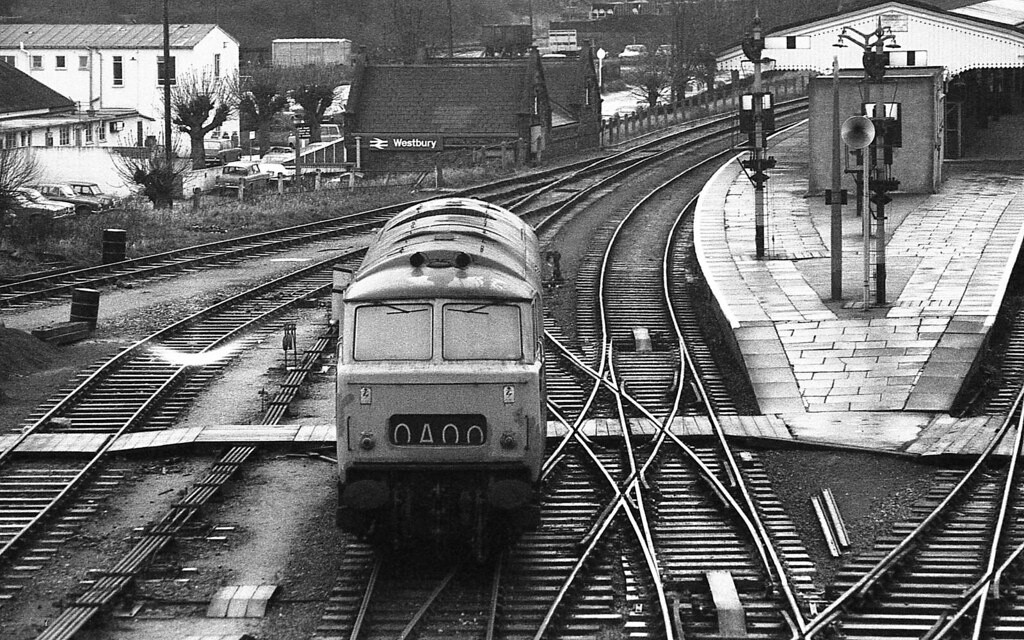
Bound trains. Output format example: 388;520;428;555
328;191;563;523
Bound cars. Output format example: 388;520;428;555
205;84;351;184
619;44;649;59
0;179;125;224
656;44;678;57
